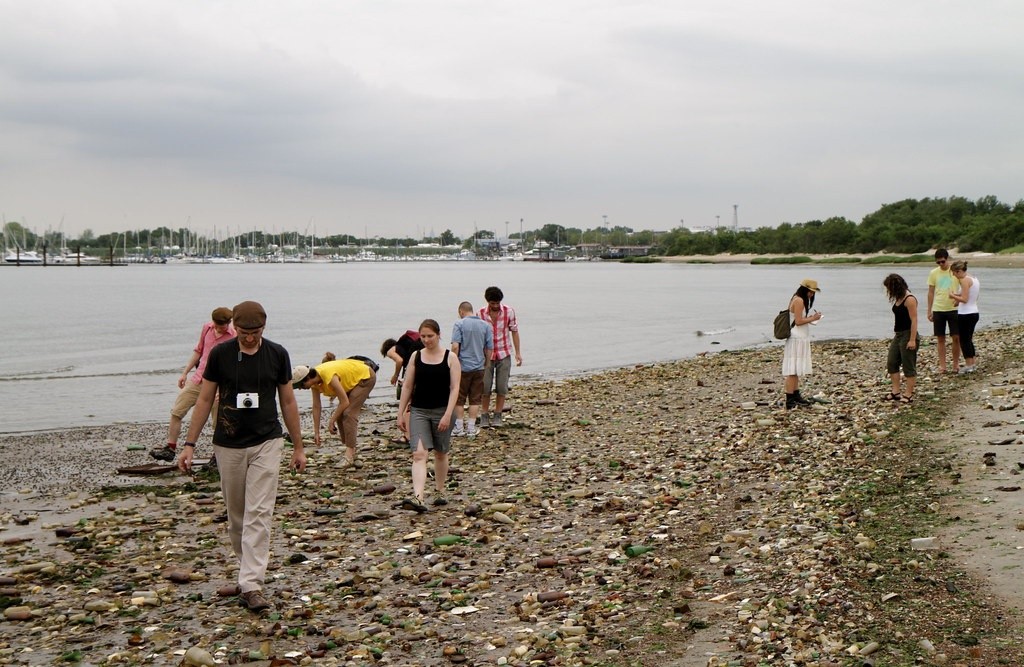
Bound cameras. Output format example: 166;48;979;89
236;393;258;408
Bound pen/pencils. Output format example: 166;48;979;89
814;309;817;312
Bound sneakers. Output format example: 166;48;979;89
466;429;479;437
491;413;502;428
434;488;448;503
240;590;270;611
480;412;490;428
150;450;175;462
958;364;977;374
402;495;427;511
451;426;463;436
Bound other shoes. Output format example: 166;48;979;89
941;370;946;374
335;457;353;468
951;370;958;374
331;451;358;462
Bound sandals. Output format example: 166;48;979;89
898;396;914;404
881;392;900;401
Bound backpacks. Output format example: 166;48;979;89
773;297;807;339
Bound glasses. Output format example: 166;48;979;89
936;259;946;265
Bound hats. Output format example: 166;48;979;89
233;302;266;330
290;364;312;383
800;278;821;293
212;308;232;323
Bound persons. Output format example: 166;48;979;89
475;287;522;428
323;351;380;403
397;318;462;512
881;273;920;403
379;330;425;446
287;360;376;469
783;279;822;409
179;300;310;611
949;260;980;373
151;308;237;462
451;302;492;437
927;249;959;374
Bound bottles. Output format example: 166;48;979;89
1;369;909;666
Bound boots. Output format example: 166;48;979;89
786;390;810;409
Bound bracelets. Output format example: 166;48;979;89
184;442;195;448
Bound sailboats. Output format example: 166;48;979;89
4;221;449;262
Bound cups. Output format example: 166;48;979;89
912;536;942;552
